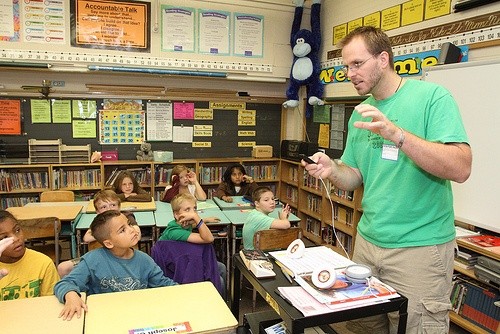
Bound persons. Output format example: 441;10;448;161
53;210;176;321
0;209;61;301
156;193;214;244
243;187;291;249
302;26;472;334
56;189;141;280
160;165;207;201
218;164;257;202
113;170;152;202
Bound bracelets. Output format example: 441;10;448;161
196;219;203;228
398;127;405;149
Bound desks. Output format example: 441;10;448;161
0;195;302;334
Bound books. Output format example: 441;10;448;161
0;167;152;202
154;165;172;185
243;165;276;195
450;227;500;334
288;165;353;255
199;163;227;199
154;188;164;201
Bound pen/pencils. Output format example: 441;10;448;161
281;267;292;283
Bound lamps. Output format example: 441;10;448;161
436;42;462;64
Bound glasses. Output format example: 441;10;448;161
340;49;382;74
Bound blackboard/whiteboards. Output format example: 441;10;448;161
422;57;499;235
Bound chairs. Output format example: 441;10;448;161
17;216;62;266
150;240;221;290
40;190;74;201
252;227;303;312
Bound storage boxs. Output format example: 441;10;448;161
258;318;283;334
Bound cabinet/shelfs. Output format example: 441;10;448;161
280;159;364;260
232;243;409;334
449;262;500;334
0;139;280;208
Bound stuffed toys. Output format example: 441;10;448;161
282;0;325;107
